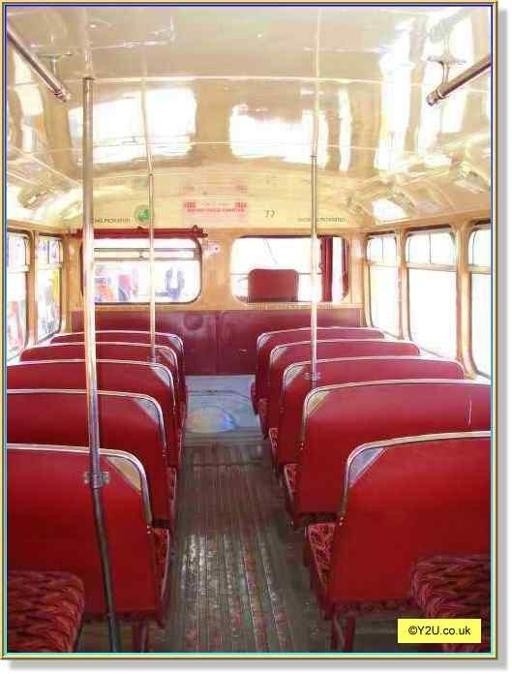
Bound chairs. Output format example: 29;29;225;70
246;268;299;302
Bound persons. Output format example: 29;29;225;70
164;261;186;301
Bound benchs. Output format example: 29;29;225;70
247;323;492;652
5;329;185;649
